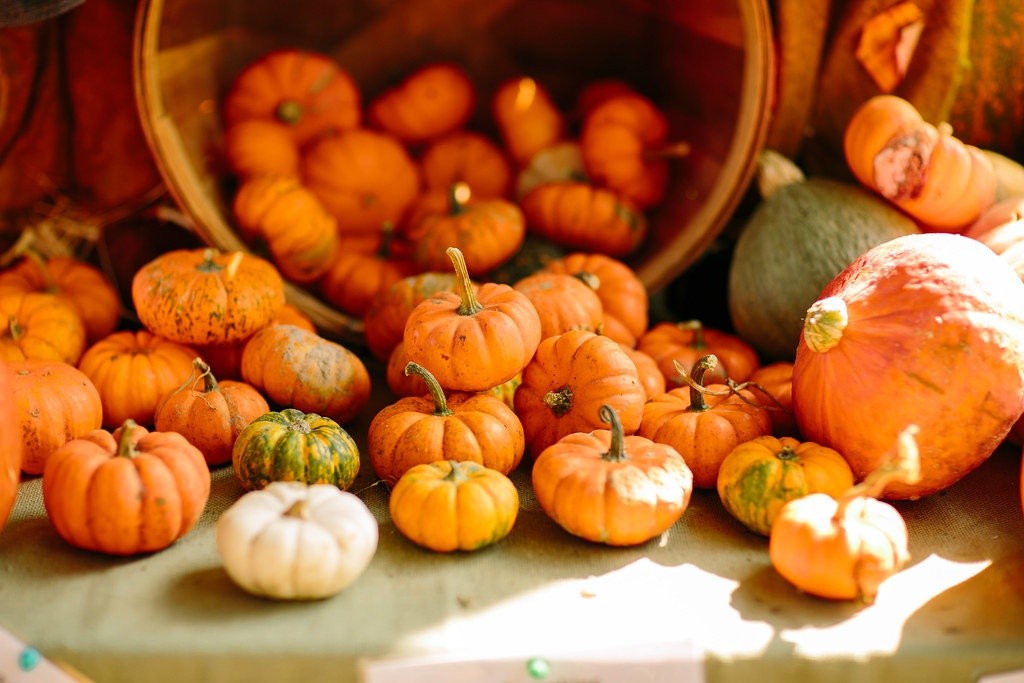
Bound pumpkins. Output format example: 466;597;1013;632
0;54;1024;603
